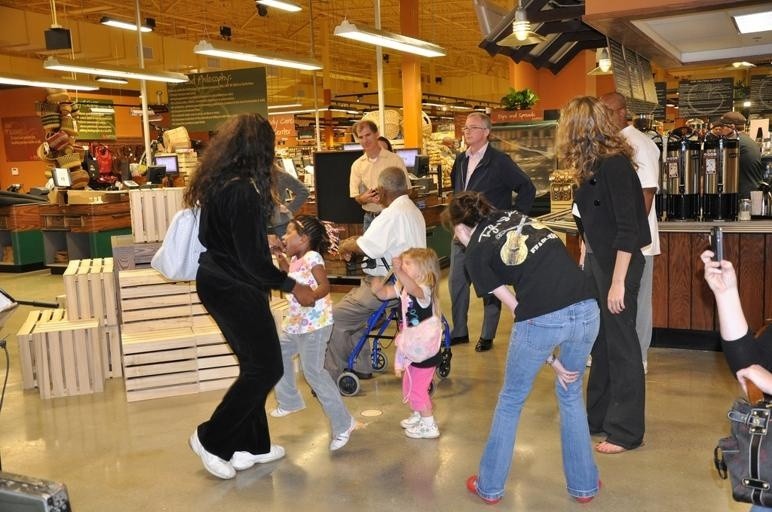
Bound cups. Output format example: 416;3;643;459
749;191;763;215
130;164;140;177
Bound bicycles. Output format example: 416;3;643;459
138;123;201;166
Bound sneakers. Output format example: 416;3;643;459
400;412;421;428
576;481;603;503
188;426;236;479
406;423;440;439
466;474;503;503
270;403;304;418
330;416;357;450
231;445;285;471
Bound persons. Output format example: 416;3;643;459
578;91;661;375
189;114;315;479
372;248;445;439
350;121;414;235
447;192;601;503
442;112;537;352
271;216;357;451
704;111;763;196
555;96;652;454
325;167;426;385
268;166;308;237
700;235;772;512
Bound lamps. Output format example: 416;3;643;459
42;1;191;85
497;1;549;47
184;0;325;77
587;45;627;76
333;1;447;59
1;71;100;91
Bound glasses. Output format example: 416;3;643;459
461;127;486;131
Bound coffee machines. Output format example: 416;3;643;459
632;112;653;136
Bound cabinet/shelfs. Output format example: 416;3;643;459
425;121;564;219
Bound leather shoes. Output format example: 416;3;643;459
442;335;470;347
475;337;493;352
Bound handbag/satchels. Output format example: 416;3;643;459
714;399;771;508
150;204;207;282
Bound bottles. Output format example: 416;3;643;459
82;145;89;160
162;176;169;187
488;131;551;150
738;199;751;222
89;195;104;204
518;157;554;191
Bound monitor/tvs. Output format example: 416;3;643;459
155;154;179;178
51;168;74;188
342;142;362;152
148;167;166;180
394;147;420;172
414;155;429;175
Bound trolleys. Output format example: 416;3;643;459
336;254;453;396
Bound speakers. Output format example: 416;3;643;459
44;29;71;50
544;109;560;120
